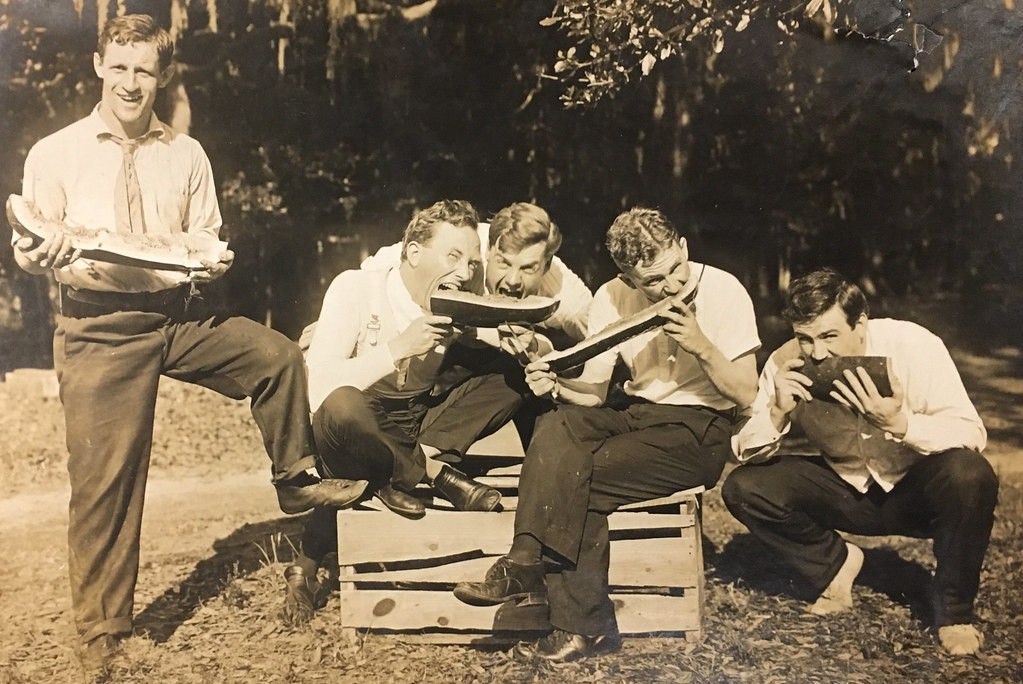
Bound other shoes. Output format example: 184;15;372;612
809;542;864;618
938;624;982;656
273;473;368;516
86;632;130;670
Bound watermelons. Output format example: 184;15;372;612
534;274;699;374
795;356;900;402
6;194;229;271
430;288;560;327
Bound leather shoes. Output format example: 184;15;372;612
453;557;549;607
430;464;502;511
367;478;426;514
513;628;621;665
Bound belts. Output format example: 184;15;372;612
66;285;186;309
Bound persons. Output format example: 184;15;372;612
720;268;998;659
306;200;528;514
365;198;590;454
9;14;370;678
453;206;761;664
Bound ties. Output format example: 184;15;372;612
105;133;150;234
